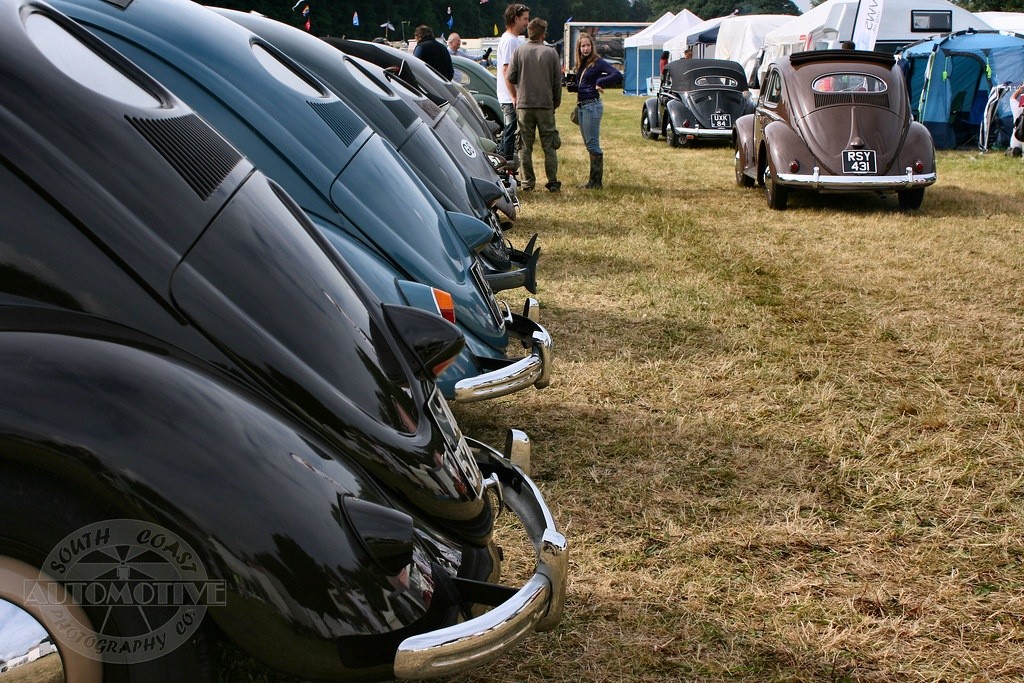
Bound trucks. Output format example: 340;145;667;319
561;21;657;90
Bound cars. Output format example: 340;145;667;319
729;46;942;214
0;4;612;683
640;57;756;150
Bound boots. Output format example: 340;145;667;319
576;153;603;189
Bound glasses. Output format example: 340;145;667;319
517;5;525;11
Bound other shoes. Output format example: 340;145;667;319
551;186;561;192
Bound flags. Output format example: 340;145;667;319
301;5;309;17
447;6;451;16
493;24;499;36
352;11;359;27
291;0;306;11
305;20;310;30
447;16;455;29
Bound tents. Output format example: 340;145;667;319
963;11;1024;36
638;8;706;97
752;0;997;139
885;28;1024;162
621;12;677;92
661;12;804;83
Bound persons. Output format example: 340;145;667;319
562;31;625;192
503;16;566;194
409;24;456;83
491;3;531;188
443;32;493;65
1008;81;1024;159
657;49;671;87
829;39;870;93
679;49;694;60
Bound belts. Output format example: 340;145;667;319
577;98;599;107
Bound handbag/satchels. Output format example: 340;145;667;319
570;106;579;125
